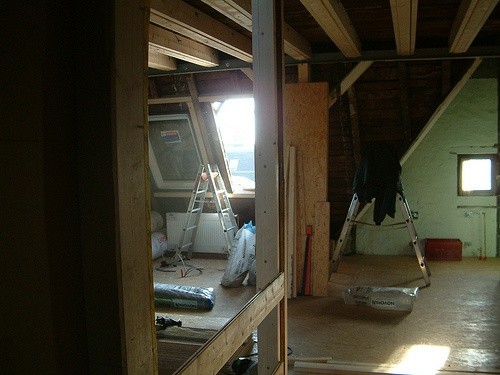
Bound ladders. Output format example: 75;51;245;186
328;162;432;287
174;163;239;262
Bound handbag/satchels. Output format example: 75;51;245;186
217;219;258;288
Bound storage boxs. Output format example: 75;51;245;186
425;237;464;262
